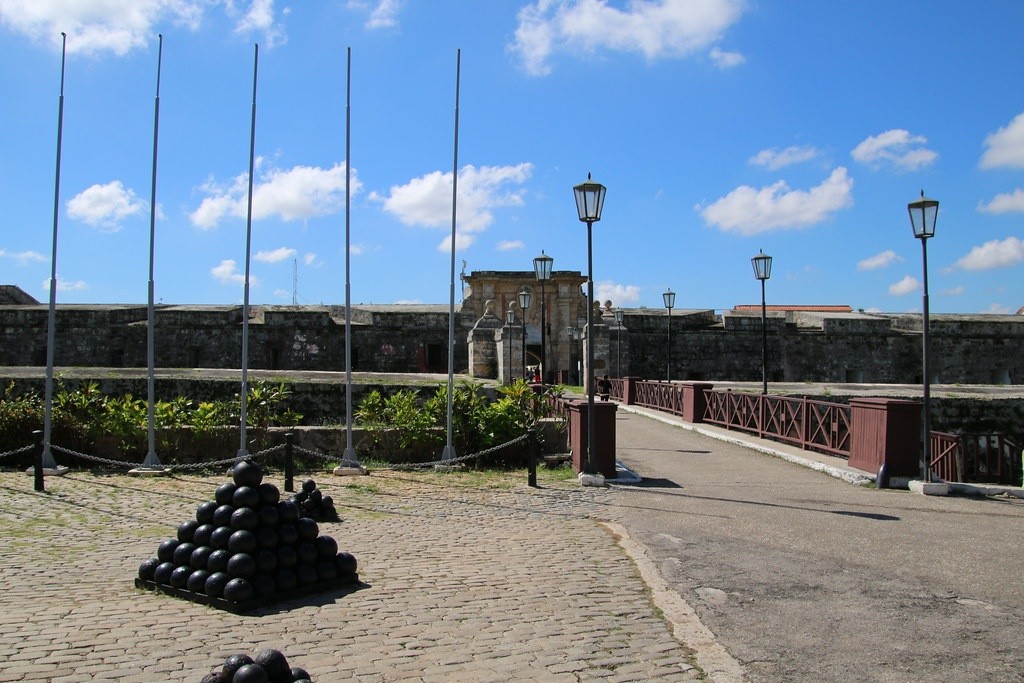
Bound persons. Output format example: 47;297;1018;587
598;375;612;402
526;366;540;384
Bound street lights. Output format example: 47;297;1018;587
532;250;553;385
908;189;940;482
750;249;772;394
517;291;531;380
663;288;675;384
505;308;515;385
572;173;607;473
612;307;624;378
